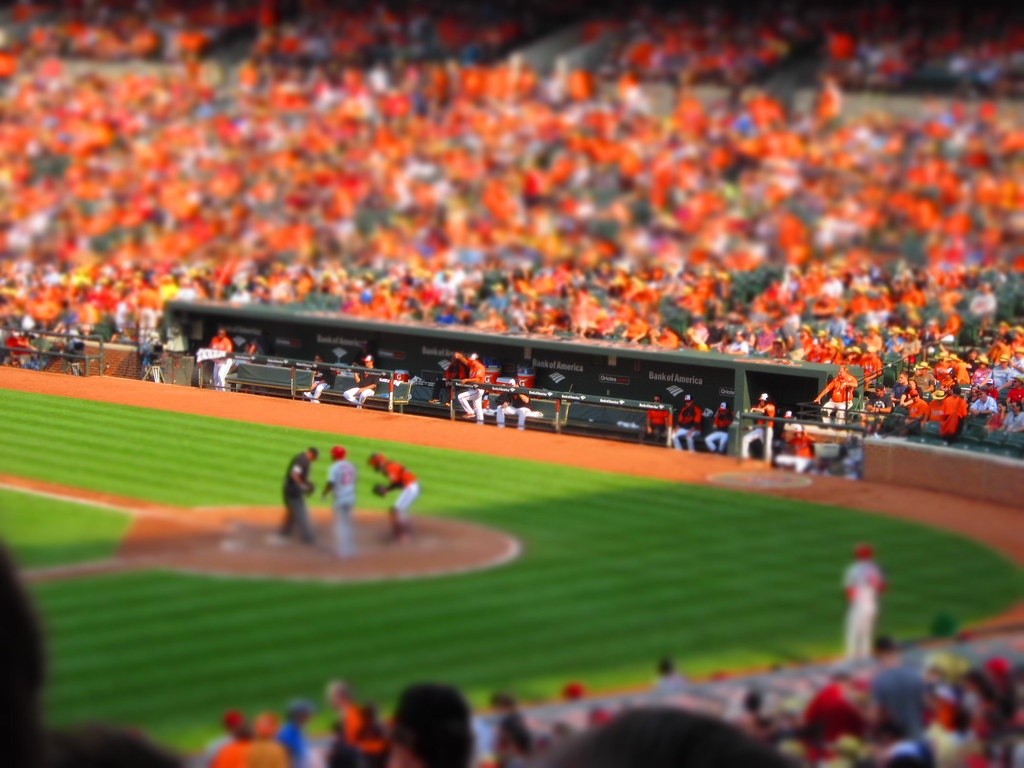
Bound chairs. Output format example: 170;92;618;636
906;416;1024;462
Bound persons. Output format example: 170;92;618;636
0;539;1024;767
322;447;365;559
0;0;1023;480
370;453;420;542
278;448;318;545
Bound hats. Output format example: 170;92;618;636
719;402;729;409
935;351;951;361
916;362;932;371
979;357;988;364
1000;354;1008;361
890;326;904;334
758;393;770;402
1015;347;1024;352
906;327;916;335
362;354;374;361
508;378;519;386
784;411;796;418
817;331;826;337
950;354;960;362
684;394;694;403
1014;326;1024;336
998;321;1008;332
310;447;318;456
470;353;478;360
802;325;811;333
794;425;805;432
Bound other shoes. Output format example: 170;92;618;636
304;392;312;398
311;399;319;403
463;414;475;418
429;399;440;402
444;402;450;407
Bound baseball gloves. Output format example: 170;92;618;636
307;480;314;499
372;481;385;497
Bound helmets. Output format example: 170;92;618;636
332;446;346;460
369;453;385;463
855;542;873;559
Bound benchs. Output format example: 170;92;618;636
225;364;572;434
